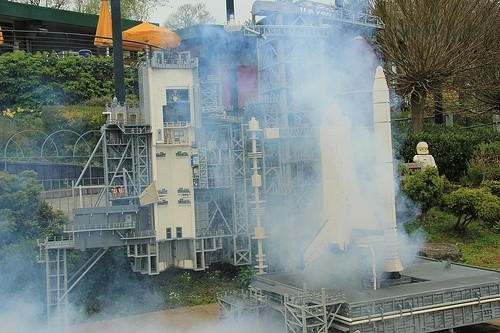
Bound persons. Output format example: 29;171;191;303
110;186;118;198
413;141;438;175
118;185;125;198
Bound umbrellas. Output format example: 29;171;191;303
121;22;181;59
94;0;114;58
